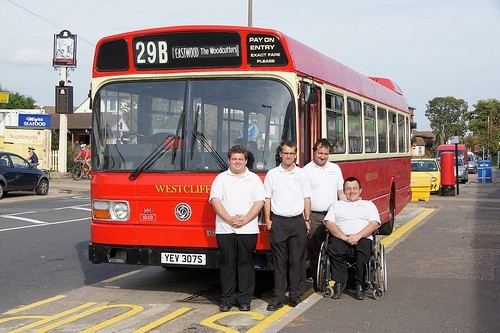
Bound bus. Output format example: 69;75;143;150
88;24;413;288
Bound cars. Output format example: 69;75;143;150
0;151;49;199
453;157;469;183
410;159;441;194
468;155;478;173
474;151;489;162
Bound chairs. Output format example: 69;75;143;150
149;124;405;157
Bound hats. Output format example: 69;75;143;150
80;144;87;148
28;146;35;151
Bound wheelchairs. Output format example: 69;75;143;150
317;229;388;301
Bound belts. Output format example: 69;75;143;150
311;211;327;215
272;214;303;219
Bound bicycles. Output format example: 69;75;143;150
72;159;92;181
24;160;49;178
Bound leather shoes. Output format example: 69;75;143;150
331;283;342;299
219;303;231;311
355;285;365;300
289;297;301;306
239;302;250;310
266;300;283;310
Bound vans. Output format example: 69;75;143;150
437;144;468;164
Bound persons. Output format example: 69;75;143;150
74;140;80;148
322;176;382;301
208;146;266;312
330;136;375;153
234;138;248;150
246;141;259;155
74;144;92;178
300;138;362;295
24;147;39;169
263;140;312;312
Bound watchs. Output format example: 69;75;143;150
305;219;312;223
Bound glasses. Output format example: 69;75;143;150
280;152;295;156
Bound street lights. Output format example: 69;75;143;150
471;113;490;159
